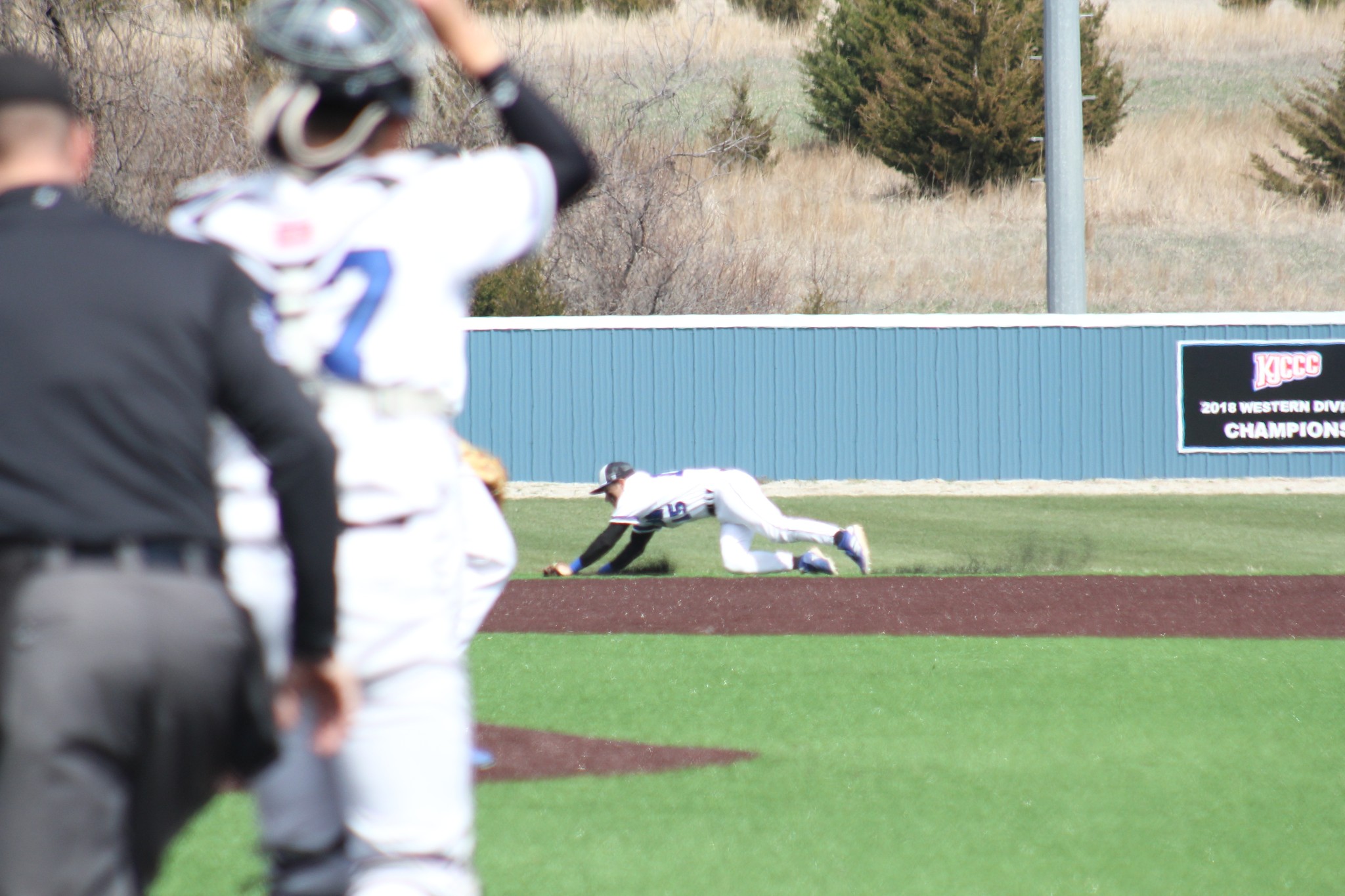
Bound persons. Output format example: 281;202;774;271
0;51;345;896
165;0;604;896
542;461;872;577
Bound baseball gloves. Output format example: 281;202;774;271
449;430;505;507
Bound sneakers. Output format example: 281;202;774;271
838;524;871;575
798;547;837;576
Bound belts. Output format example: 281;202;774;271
17;539;227;576
704;468;728;517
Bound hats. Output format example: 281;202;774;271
589;461;634;495
0;53;76;109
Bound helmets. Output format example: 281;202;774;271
245;0;421;92
